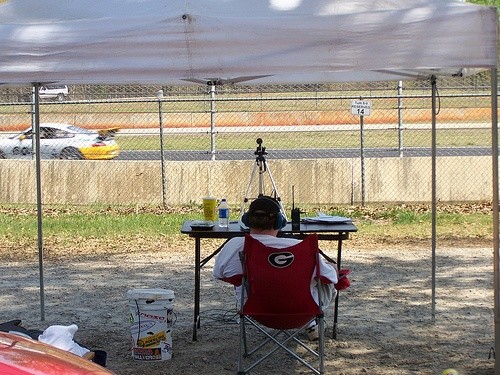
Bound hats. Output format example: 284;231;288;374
249;196;280;218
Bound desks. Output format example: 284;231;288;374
181;221;358;341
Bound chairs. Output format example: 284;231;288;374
216;226;351;375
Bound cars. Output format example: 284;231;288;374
0;123;120;160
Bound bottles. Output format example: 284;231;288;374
218;198;230;227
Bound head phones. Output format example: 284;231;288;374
241;197;286;229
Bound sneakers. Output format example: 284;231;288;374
305;319;326;340
258;323;274;334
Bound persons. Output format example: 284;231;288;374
213;195;339;342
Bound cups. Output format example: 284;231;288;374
201;196;217;223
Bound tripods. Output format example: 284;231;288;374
239;155;288;222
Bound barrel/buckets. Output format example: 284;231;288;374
126;288;177;363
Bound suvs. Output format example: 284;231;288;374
38;85;69;101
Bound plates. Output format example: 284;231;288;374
190;221;215;229
